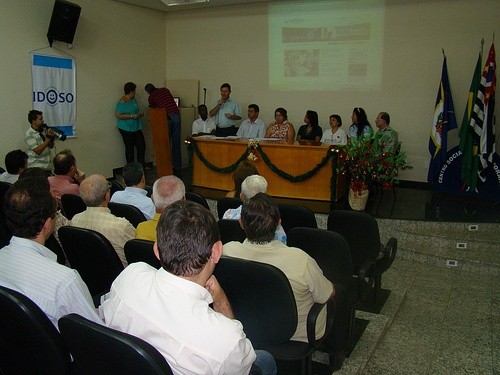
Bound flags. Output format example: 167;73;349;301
428;45;500;201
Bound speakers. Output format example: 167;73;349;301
47;0;81;44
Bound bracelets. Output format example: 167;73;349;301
131;115;132;119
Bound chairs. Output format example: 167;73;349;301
0;168;397;375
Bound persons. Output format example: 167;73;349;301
115;82;146;163
48;150;85;206
110;162;156;220
25;110;56;177
236;104;294;138
297;107;397;200
209;83;242;136
223;175;288;245
223;193;352;370
145;83;183;175
69;174;136;268
188;104;217;163
102;200;278;375
19;168;70;258
134;175;185;240
0;177;105;362
226;162;259;199
0;150;29;184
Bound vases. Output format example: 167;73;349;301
348;188;369;211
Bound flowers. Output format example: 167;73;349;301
335;127;414;197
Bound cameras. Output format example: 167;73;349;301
39;125;67;142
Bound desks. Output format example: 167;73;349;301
183;137;346;202
